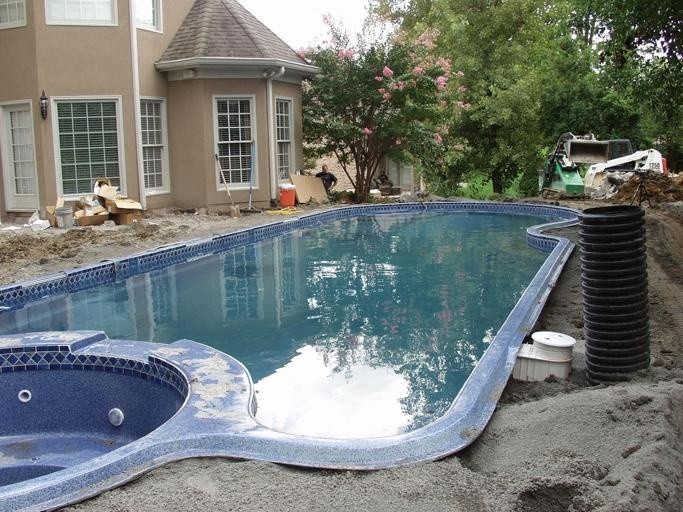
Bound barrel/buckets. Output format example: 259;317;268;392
278;187;297;209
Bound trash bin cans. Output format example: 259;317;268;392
57;208;73;229
278;184;297;208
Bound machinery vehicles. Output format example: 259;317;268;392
537;132;680;203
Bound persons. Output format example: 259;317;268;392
315;164;338;196
377;171;389;184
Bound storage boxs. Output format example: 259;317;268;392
24;183;140;232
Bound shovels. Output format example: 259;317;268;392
215;154;241;217
